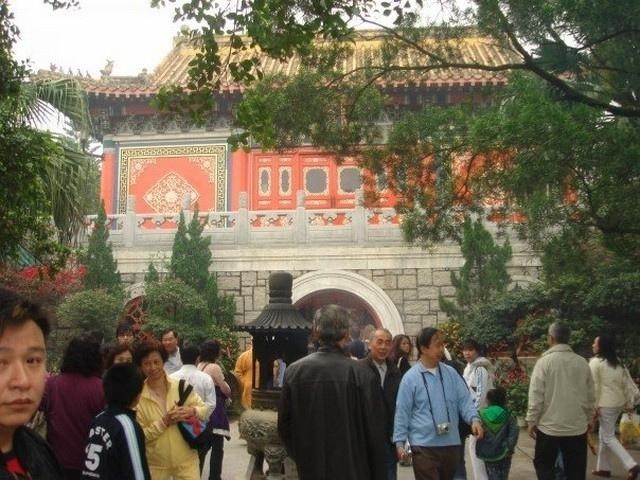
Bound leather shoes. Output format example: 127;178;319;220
592;470;610;476
627;465;640;480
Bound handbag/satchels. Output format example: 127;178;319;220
621;411;640;448
177;416;212;449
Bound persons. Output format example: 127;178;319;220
277;301;415;480
475;389;519;480
41;325;231;480
234;336;261;409
461;339;495;480
1;286;65;480
589;334;639;480
527;320;596;478
394;327;483;480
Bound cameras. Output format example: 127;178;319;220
437;422;451;434
